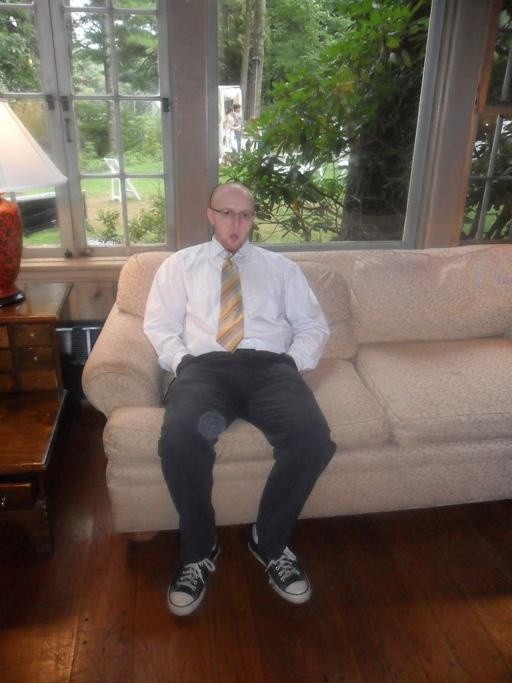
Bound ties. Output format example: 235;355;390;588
216;254;245;353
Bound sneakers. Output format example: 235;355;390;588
167;532;222;617
246;523;312;605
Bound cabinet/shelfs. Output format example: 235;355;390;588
0;280;75;557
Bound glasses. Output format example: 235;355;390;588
209;206;254;220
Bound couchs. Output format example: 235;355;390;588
80;243;512;541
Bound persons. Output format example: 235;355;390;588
142;180;334;617
225;106;240;150
233;103;243;152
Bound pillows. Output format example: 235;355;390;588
294;246;512;360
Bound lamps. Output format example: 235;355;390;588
1;100;69;311
